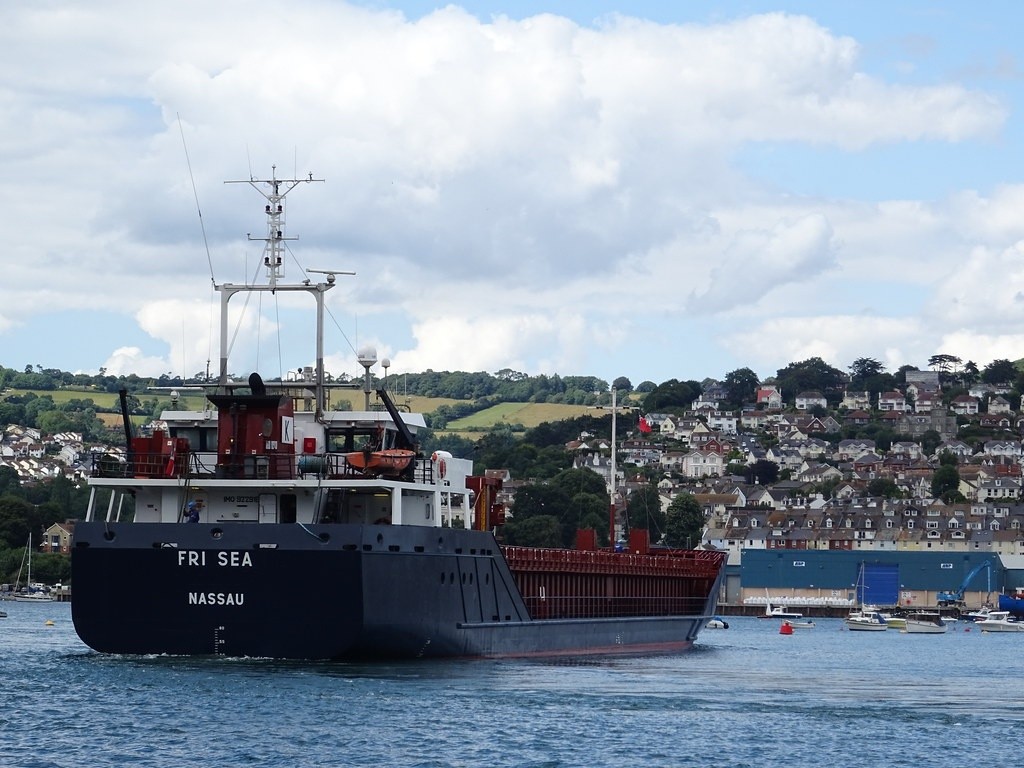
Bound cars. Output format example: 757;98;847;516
0;581;71;594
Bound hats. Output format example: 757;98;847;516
189;501;197;509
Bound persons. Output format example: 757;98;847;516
808;619;812;624
185;499;200;524
614;541;624;554
953;627;955;630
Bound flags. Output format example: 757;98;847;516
638;417;652;433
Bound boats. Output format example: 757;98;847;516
778;621;795;634
61;108;730;668
845;560;1023;627
976;611;1024;633
703;618;728;629
780;618;816;628
0;611;7;618
904;613;948;633
847;619;888;630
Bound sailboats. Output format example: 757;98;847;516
12;532;54;601
755;584;804;618
